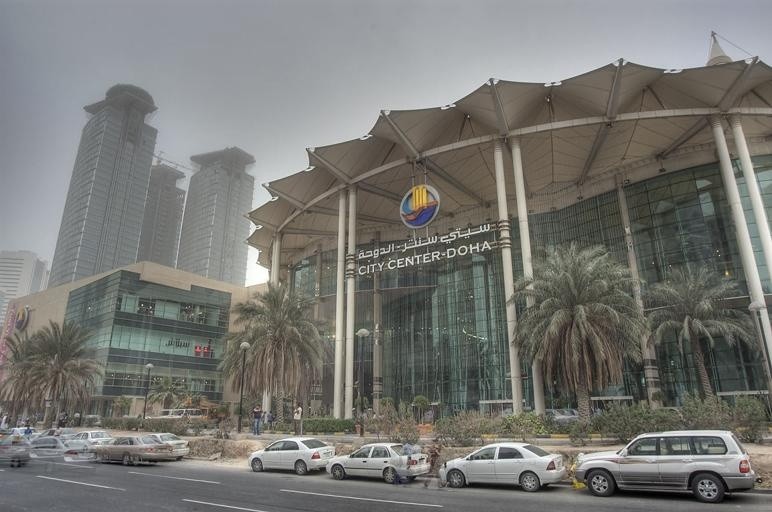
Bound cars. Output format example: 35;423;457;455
0;427;190;468
160;409;204;424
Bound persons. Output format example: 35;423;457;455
57;408;68;428
24;426;32;435
253;404;273;435
293;401;302;435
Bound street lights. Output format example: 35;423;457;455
237;342;251;433
142;363;154;428
356;329;369;437
748;300;771;392
